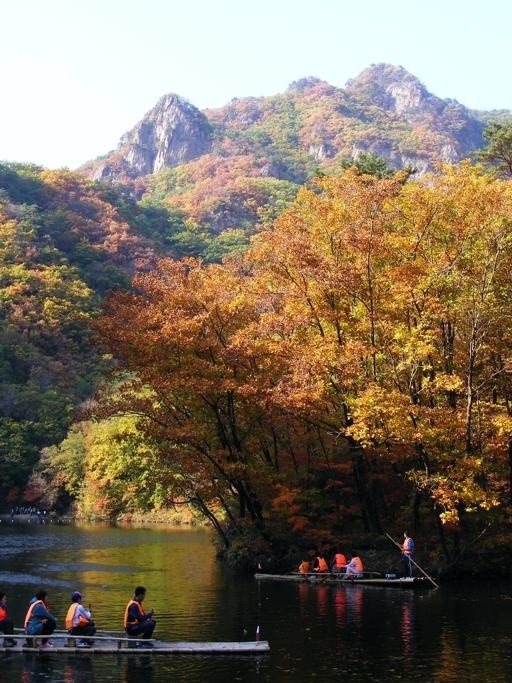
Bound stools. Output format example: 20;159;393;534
125;634;138;648
63;634;76;647
22;635;39;648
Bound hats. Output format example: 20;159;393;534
72;591;85;600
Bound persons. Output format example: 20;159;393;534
65;591;96;648
0;591;18;647
257;559;267;573
24;590;57;648
399;531;414;580
298;550;364;579
12;505;47;518
124;587;156;648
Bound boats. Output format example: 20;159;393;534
255;572;435;583
0;627;270;651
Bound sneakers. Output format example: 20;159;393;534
77;640;94;647
3;640;17;647
140;642;154;648
40;641;56;648
